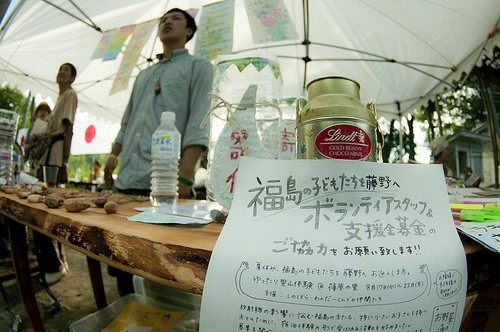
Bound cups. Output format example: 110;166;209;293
43;166;59;192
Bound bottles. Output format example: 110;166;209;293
10;149;16;186
75;167;81;182
150;111;182;213
0;108;19;188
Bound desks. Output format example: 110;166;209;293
0;189;478;332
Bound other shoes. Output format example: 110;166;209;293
63;261;69;272
39;268;66;284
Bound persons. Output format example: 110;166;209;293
104;8;214;298
24;103;52;182
30;63;78;285
87;160;105;192
427;135;452;176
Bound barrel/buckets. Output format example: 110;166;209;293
297;76;377;162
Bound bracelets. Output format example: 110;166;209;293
177;173;194;186
108;154;118;170
